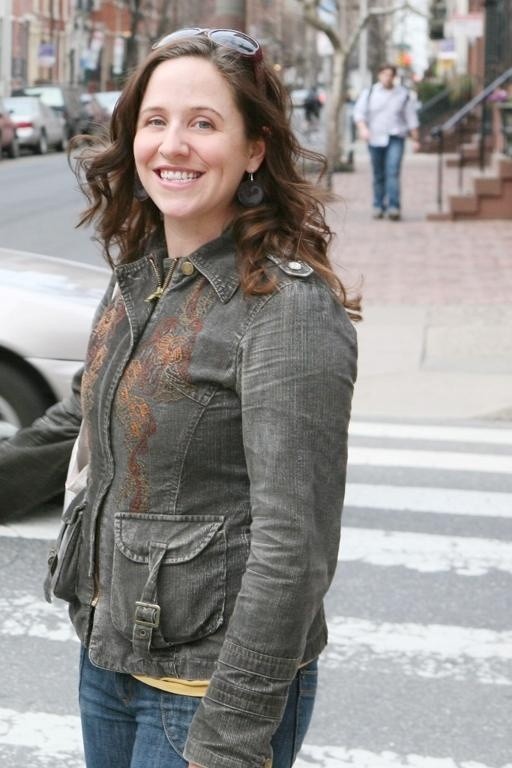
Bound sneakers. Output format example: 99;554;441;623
372;207;399;221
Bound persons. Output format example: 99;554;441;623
353;63;421;220
303;83;322;121
2;27;357;768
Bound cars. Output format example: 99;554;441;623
1;340;86;520
1;88;120;157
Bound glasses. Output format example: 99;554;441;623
151;27;265;89
